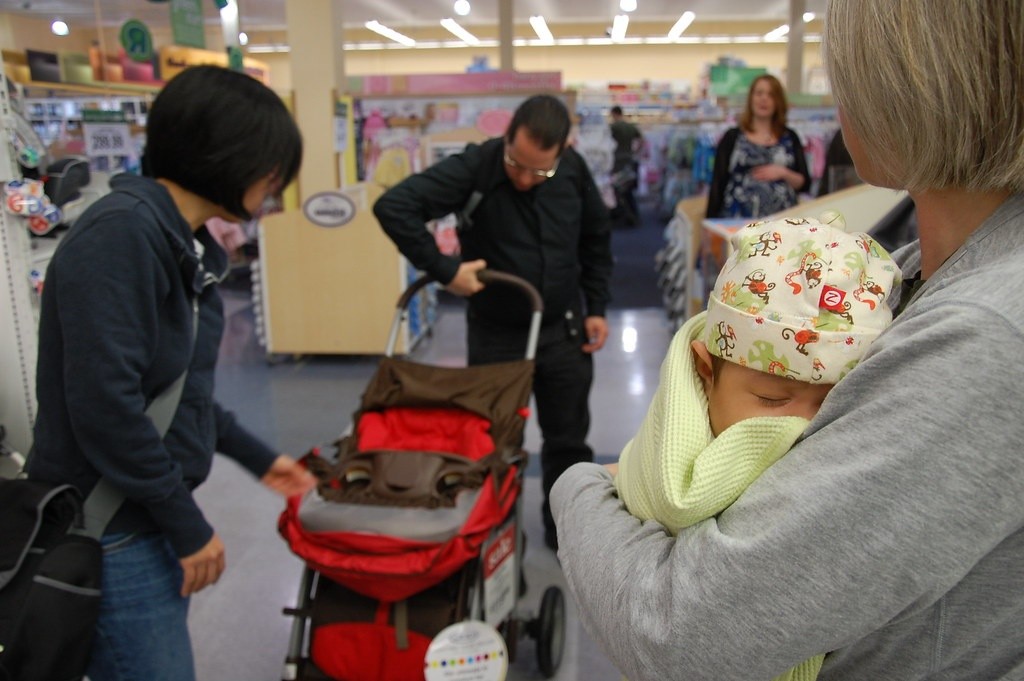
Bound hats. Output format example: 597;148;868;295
703;207;903;381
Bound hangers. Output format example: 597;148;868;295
696;108;840;148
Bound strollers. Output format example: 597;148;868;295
276;268;567;681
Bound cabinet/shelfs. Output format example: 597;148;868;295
25;96;156;148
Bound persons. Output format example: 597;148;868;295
705;75;812;219
610;106;644;228
549;1;1024;681
26;65;318;681
372;96;613;598
614;218;903;537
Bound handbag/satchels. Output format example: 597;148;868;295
1;475;106;681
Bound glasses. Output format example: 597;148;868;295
503;141;563;177
